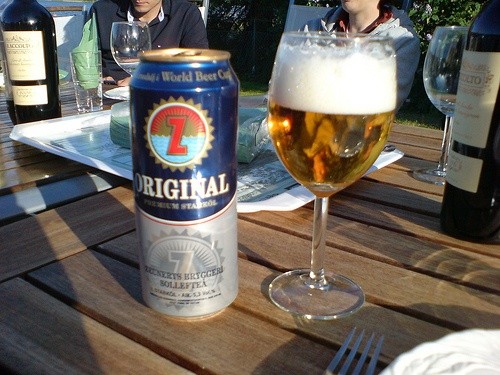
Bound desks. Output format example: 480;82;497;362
0;81;500;374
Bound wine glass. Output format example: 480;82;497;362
110;21;151;79
412;27;470;185
266;30;399;319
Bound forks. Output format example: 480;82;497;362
322;326;385;375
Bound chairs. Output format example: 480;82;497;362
41;5;88;82
284;1;410;32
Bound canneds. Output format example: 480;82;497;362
129;47;238;321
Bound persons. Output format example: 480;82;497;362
86;0;209;86
297;0;420;114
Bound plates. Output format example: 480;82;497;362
379;329;500;375
104;86;130;101
7;109;405;214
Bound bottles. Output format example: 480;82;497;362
1;1;62;125
440;0;500;246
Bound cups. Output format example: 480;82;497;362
68;49;103;113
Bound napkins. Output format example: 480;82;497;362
379;328;500;374
111;102;269;163
72;13;101;88
